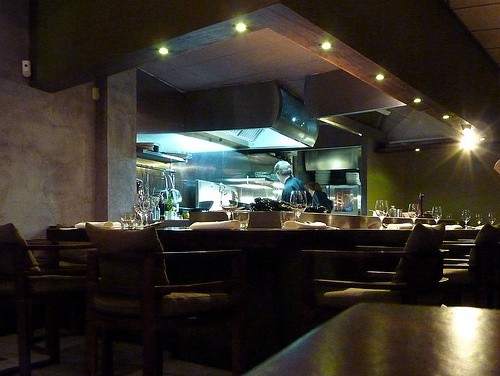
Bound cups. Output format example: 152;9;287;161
119;213;136;230
279;211;294;230
447;213;453;219
232;210;249;229
396;209;402;217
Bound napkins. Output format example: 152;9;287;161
472;225;484;230
282;220;341;231
445;224;464;231
74;222;115;230
188;219;241;231
386;223;430;231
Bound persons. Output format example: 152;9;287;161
308;182;333;214
274;160;312;206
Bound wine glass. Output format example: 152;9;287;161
461;210;470;229
375;200;388;231
220;188;238;221
408;203;419;229
134;194;154;228
431;206;442;226
488;212;496;226
475;213;483;227
290;190;307;223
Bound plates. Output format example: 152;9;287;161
136;142;160;153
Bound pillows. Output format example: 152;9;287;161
467;223;499;285
85;223;174;294
390;223;445;289
0;222;42;279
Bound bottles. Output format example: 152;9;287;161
155;194;173;221
389;205;396;217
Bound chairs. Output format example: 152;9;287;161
0;222;500;376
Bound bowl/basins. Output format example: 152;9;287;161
313;169;360;186
